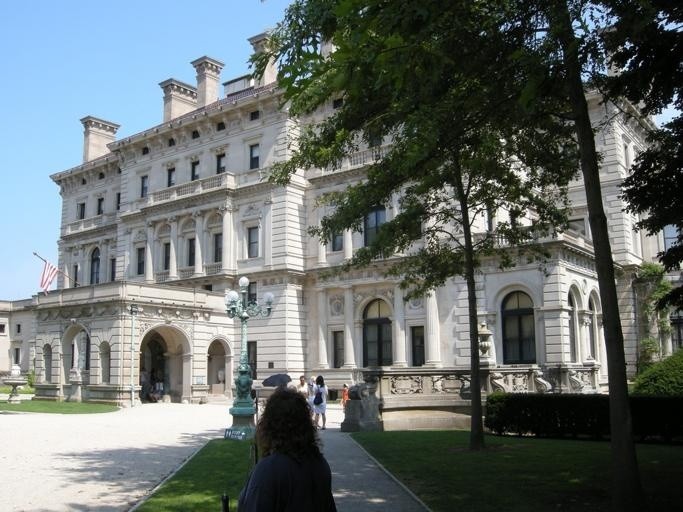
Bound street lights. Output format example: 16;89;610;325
224;274;274;407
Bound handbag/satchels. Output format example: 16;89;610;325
314;385;323;405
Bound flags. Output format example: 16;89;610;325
39;262;58;296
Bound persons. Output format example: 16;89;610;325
237;387;335;511
310;374;329;430
139;367;162;402
295;375;308;398
339;382;349;408
